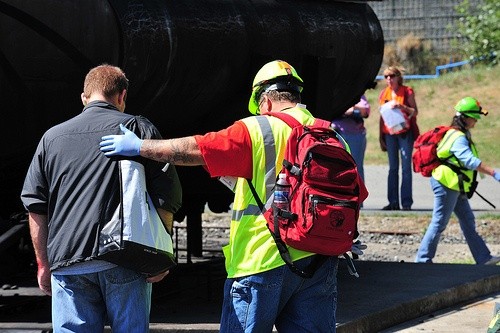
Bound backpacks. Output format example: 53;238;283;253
245;112;361;277
412;125;471;177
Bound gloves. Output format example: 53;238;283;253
99;124;144;157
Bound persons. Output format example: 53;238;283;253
415;97;500;265
330;94;370;209
99;60;351;333
379;66;418;210
20;65;183;333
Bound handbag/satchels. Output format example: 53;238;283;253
96;116;177;276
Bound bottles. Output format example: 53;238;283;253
274;174;290;223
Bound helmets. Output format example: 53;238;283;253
249;60;303;116
454;96;481;119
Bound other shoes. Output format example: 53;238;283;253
484;256;500;265
382;204;400;210
402;206;411;210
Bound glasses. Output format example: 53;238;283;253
384;74;398;79
255;96;265;115
